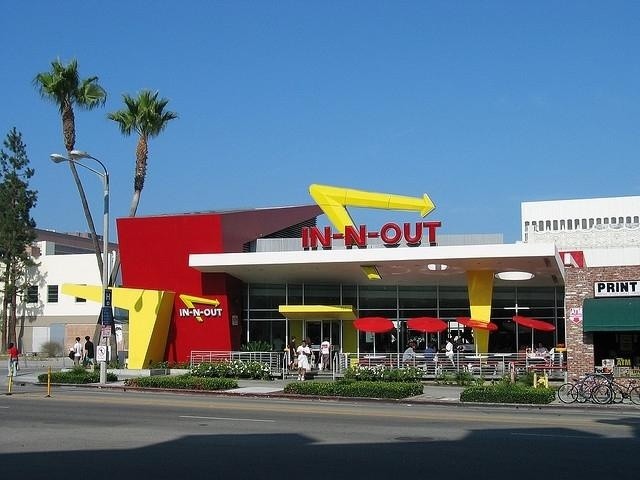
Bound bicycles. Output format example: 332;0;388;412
557;371;640;406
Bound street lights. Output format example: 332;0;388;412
52;148;110;383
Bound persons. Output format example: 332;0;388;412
319;337;331;372
304;337;313;354
6;343;19;377
536;342;550;353
70;337;82;366
84;336;94;364
296;339;312;381
402;337;456;375
289;335;296;371
